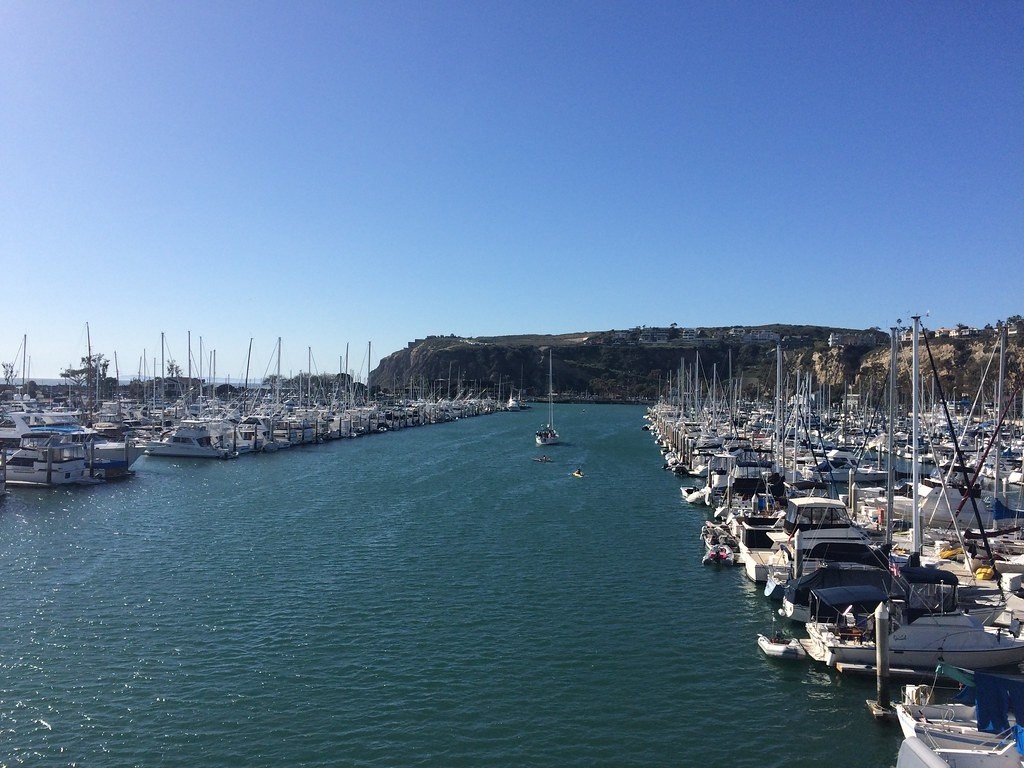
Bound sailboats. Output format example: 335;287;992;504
643;310;1024;768
0;323;531;487
536;350;559;445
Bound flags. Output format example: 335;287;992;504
889;555;902;577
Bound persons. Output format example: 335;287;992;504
536;430;554;438
576;468;580;474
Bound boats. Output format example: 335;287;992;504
533;455;550;462
572;472;584;477
